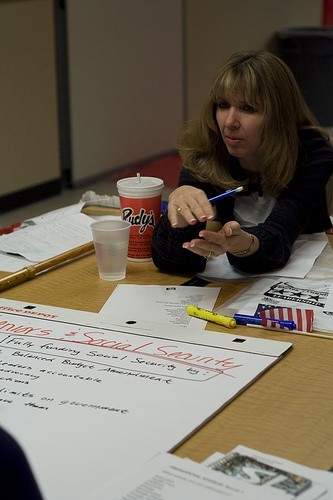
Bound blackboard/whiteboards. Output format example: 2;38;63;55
60;0;189;193
0;0;67;215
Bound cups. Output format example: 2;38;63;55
117;177;165;262
90;219;132;281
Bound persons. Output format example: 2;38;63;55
151;50;333;276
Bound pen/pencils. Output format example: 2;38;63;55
207;186;244;202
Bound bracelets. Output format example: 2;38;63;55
232;233;255;256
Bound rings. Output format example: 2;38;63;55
204;252;212;261
177;206;189;214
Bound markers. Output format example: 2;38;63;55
186;305;237;330
232;313;297;330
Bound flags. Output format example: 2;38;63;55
254;304;314;333
